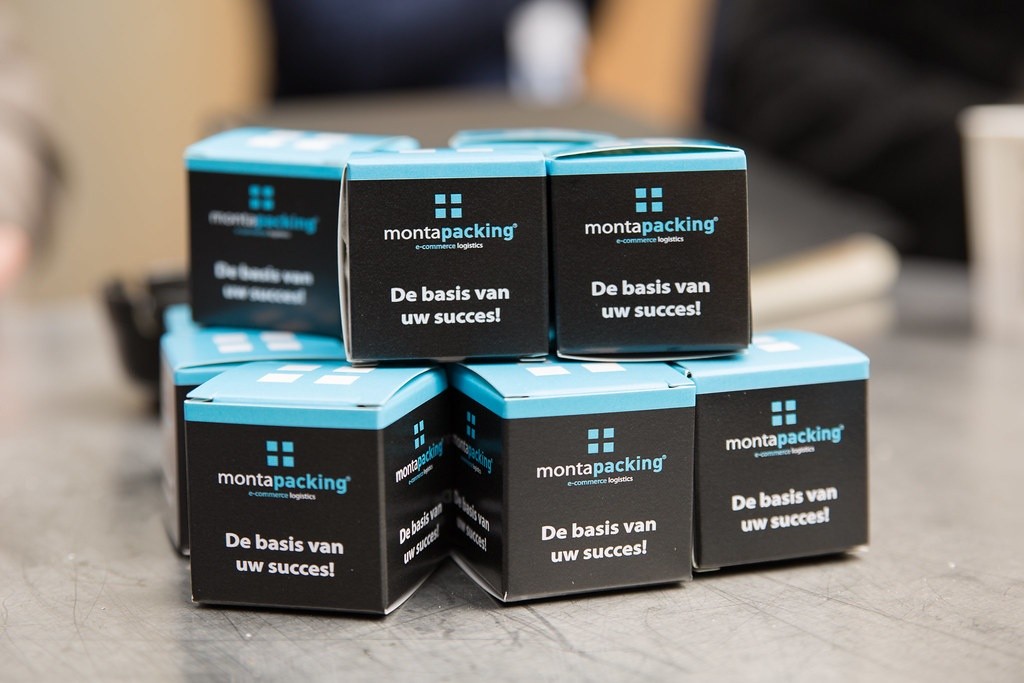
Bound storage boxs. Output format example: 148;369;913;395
159;125;871;612
